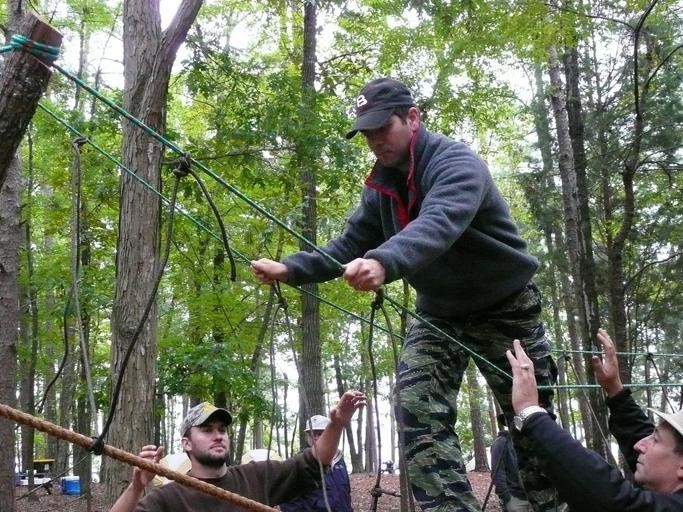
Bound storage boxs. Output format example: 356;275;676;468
61;476;80;495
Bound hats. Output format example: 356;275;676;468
303;413;333;434
649;402;683;438
344;78;415;140
180;401;234;440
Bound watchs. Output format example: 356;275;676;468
513;406;548;432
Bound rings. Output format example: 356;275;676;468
520;365;530;369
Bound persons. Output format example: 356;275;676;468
280;415;354;512
506;328;683;512
110;390;368;512
249;79;571;512
490;431;535;512
